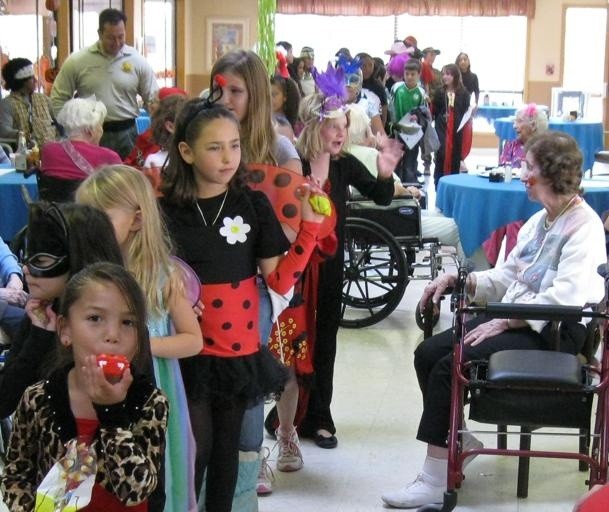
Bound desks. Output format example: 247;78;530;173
436;172;609;257
494;118;604;179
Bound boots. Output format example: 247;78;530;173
232;450;260;511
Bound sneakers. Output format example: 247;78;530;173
383;478;448;506
313;430;338;447
276;427;303;470
461;431;483;469
257;449;275;495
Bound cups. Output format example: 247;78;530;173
505;162;512;183
32;147;39;160
26;150;32;162
10;153;15;167
460;161;467;173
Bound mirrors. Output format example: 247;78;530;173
0;15;51;100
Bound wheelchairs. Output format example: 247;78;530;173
338;183;460;329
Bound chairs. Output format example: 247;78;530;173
590;131;609;178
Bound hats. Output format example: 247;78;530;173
384;41;415;56
398;36;419;50
423;47;440;56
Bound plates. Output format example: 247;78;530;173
511;176;521;179
478;173;489;177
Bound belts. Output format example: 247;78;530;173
104;118;135;131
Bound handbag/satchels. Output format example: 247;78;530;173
424;109;440;156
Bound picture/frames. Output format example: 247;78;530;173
206;16;248;72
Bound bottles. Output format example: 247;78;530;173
15;131;27;173
503;162;512;183
32;144;39;164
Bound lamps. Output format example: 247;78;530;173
51;35;57;60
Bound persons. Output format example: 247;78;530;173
301;47;320;95
374;63;390;100
0;236;27;351
0;263;169;511
210;49;303;512
258;117;335;495
343;102;467;266
389;59;426;186
501;104;548;170
456;52;479;160
1;202;157;421
269;75;299;131
381;130;607;508
423;48;443;100
42;96;120;186
334;59;387;137
355;54;388;129
275;41;294;78
145;93;188;172
429;64;471;190
50;9;159;159
287;57;305;98
73;163;204;511
385;53;410;90
405;36;417;58
265;93;404;448
1;58;60;152
160;97;330;512
387;43;406;61
